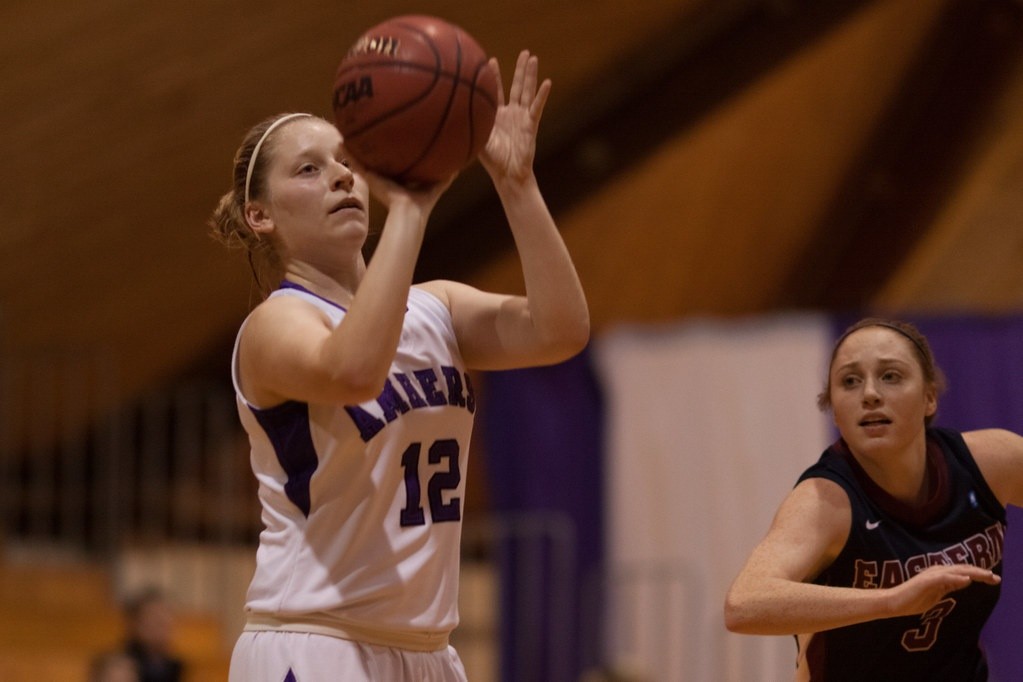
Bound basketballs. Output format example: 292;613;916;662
332;12;501;193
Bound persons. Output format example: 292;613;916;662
725;318;1023;682
210;50;590;682
